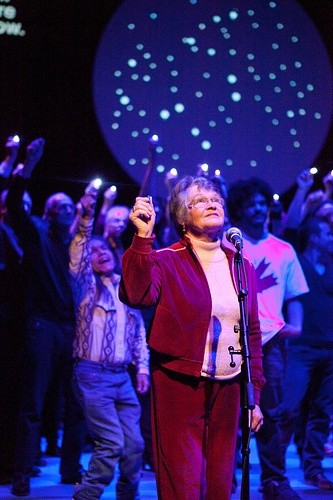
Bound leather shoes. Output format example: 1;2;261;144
305;472;333;489
61;468;88;483
13;475;30;495
263;483;302;500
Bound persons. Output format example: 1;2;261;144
0;134;180;496
226;178;309;500
270;167;333;500
119;177;265;500
67;180;151;500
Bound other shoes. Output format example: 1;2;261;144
237;462;253;469
29;472;39;476
84;444;92;452
0;471;14;483
324;444;333;455
34;460;46;466
46;447;62;457
232;481;237;493
32;468;40;473
259;486;264;491
141;461;156;476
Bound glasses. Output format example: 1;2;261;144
183;198;225;216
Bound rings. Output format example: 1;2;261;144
132;206;135;212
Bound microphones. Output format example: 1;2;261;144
226;226;243;250
